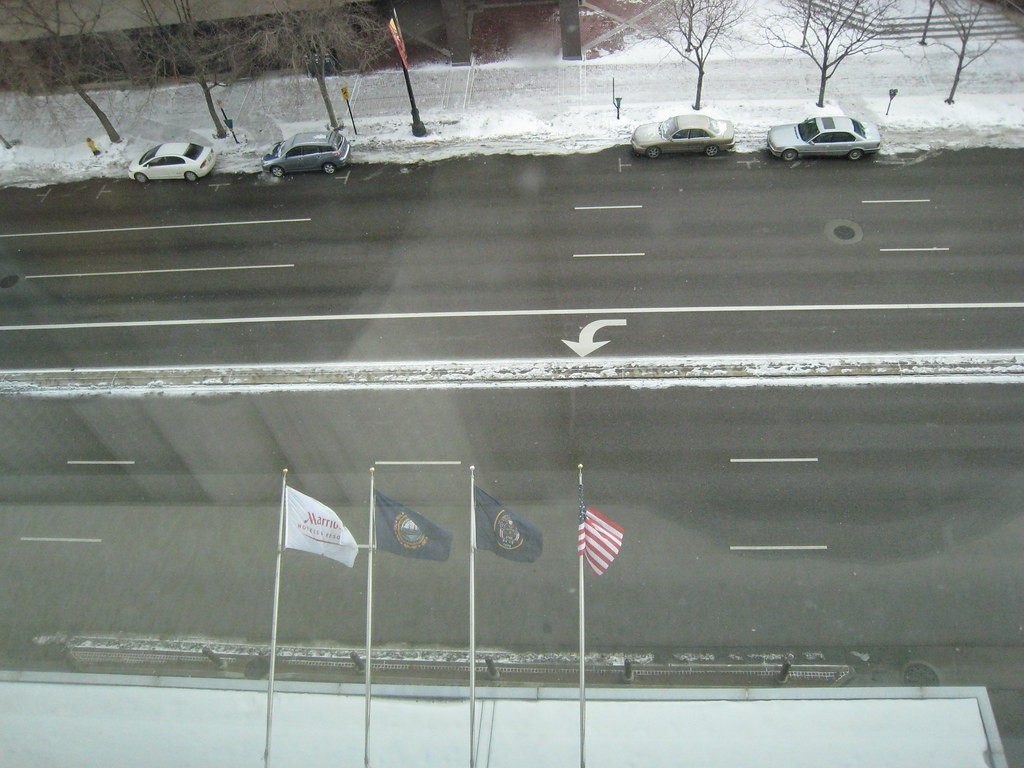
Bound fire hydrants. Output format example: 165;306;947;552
87;137;100;155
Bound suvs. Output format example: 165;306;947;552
261;131;352;177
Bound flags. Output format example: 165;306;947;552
474;485;542;562
373;488;451;561
577;484;624;577
284;485;359;569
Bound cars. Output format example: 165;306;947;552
631;114;735;159
128;142;216;184
767;116;881;162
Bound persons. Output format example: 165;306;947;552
87;138;100;155
